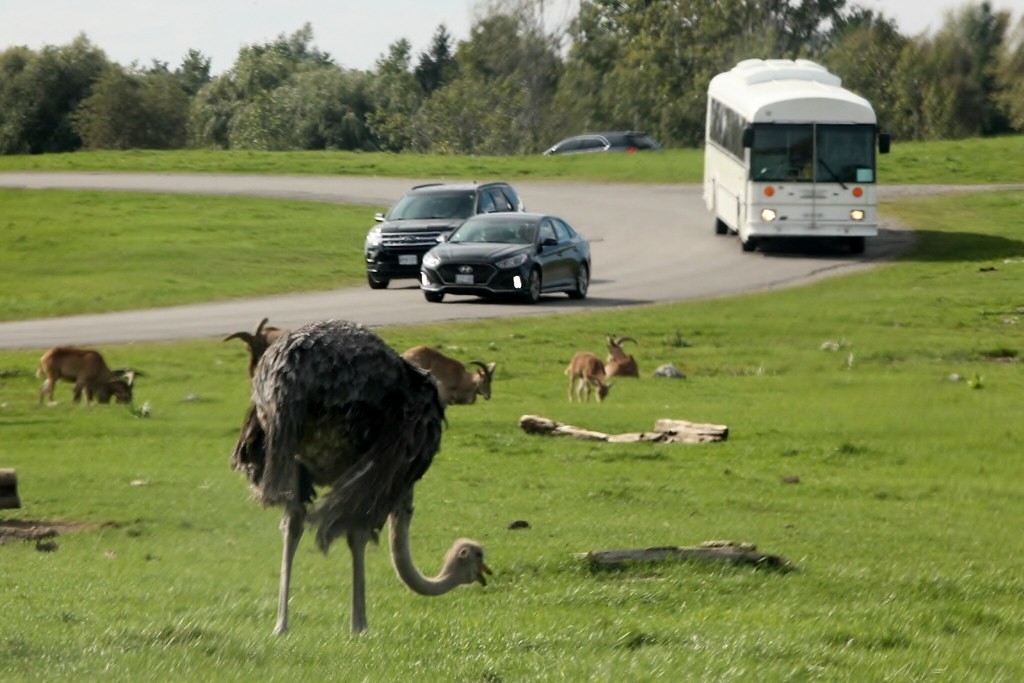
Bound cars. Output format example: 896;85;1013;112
420;210;592;306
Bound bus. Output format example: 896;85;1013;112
702;57;891;255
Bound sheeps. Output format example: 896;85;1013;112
604;336;639;376
36;346;133;405
224;317;281;378
566;351;616;403
401;346;497;405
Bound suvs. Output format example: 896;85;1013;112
541;130;660;155
363;181;526;290
